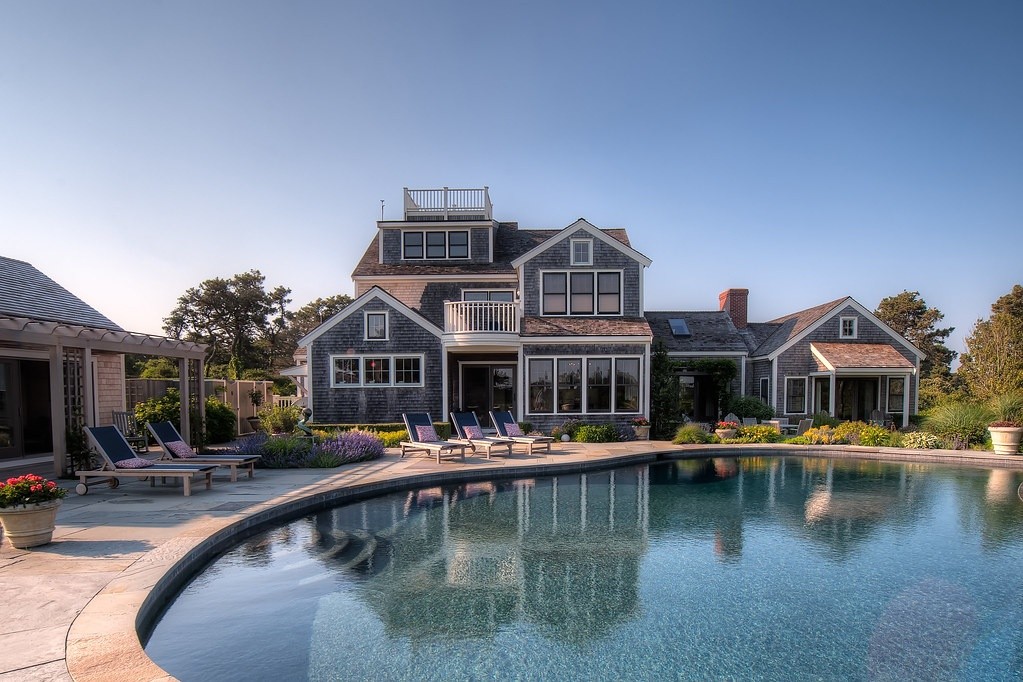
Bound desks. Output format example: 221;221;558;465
779;424;799;436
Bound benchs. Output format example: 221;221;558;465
74;425;222;498
772;418;791;435
139;420;262;483
762;420;788;436
398;412;471;464
489;411;555;455
447;412;516;459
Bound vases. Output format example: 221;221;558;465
632;425;651;440
0;498;64;549
561;404;570;410
716;429;738;440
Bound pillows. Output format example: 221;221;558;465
462;425;485;439
504;421;524;437
415;425;438;442
116;458;152;469
165;440;199;460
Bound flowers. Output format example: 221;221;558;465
718;421;738;429
0;473;69;509
632;417;651;427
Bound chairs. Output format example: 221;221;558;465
111;408;148;452
743;417;758;425
791;418;814;434
789;420;812;438
